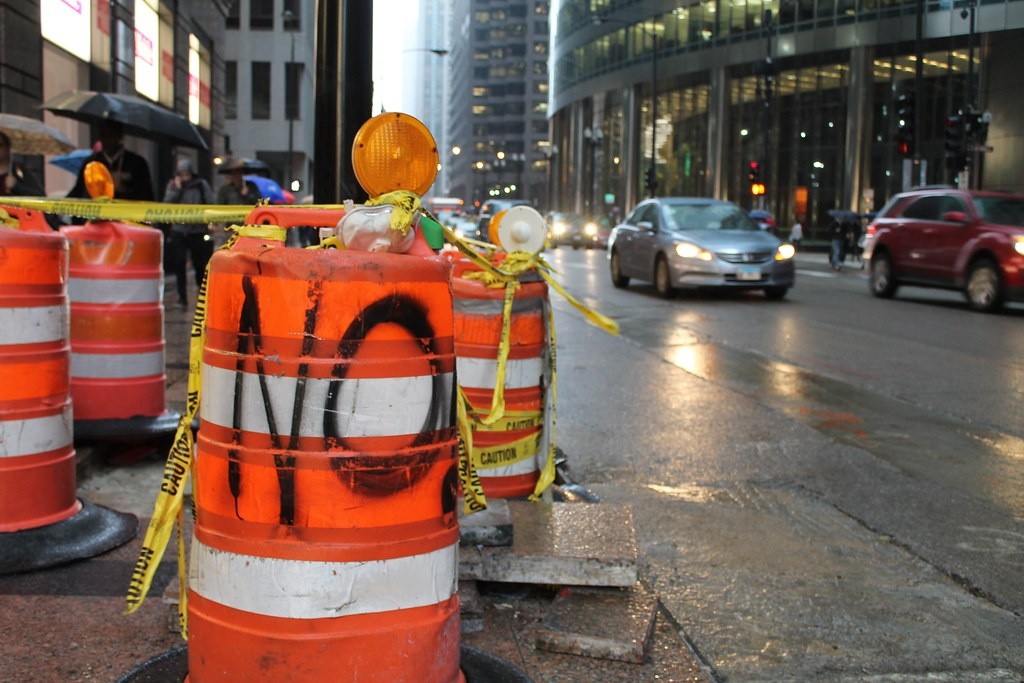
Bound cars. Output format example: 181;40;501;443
607;197;798;300
423;197;537;244
542;211;615;249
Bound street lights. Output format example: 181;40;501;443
380;49;450;113
592;16;659;200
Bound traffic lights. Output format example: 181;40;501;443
644;167;654;190
750;161;759;179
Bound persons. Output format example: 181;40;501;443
218;170;258;244
64;119;155;228
767;216;776;234
0;132;48;197
827;218;876;272
163;160;216;310
788;220;803;253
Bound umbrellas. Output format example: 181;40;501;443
827;210;878;217
747;209;769;218
272;189;296;205
242;176;286;205
49;149;93;176
0;113;80;158
218;157;272;176
45;89;209;150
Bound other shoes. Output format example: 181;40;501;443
173;300;188;310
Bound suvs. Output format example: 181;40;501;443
864;185;1024;311
